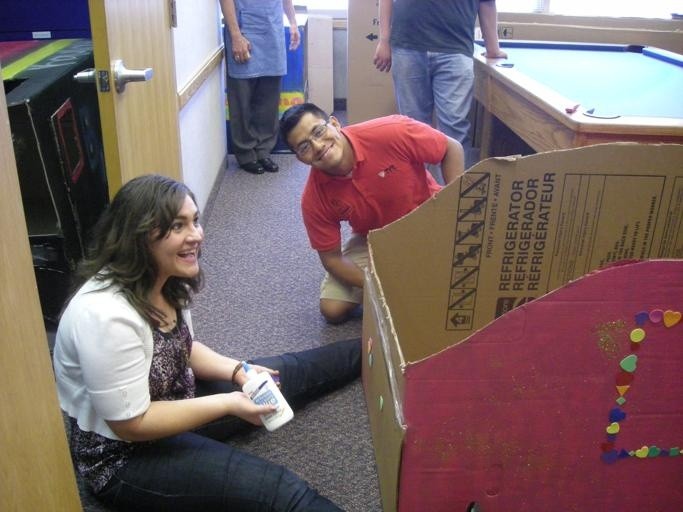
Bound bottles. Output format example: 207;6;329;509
240;359;295;433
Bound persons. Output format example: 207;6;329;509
373;0;508;187
276;102;464;324
50;175;363;512
220;0;300;173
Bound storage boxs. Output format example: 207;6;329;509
361;142;683;512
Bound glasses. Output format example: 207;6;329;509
296;122;327;155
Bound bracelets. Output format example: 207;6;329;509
230;361;247;385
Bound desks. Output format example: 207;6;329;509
472;39;683;163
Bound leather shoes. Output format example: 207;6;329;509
240;158;279;174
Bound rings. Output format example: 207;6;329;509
243;52;250;58
234;55;239;60
